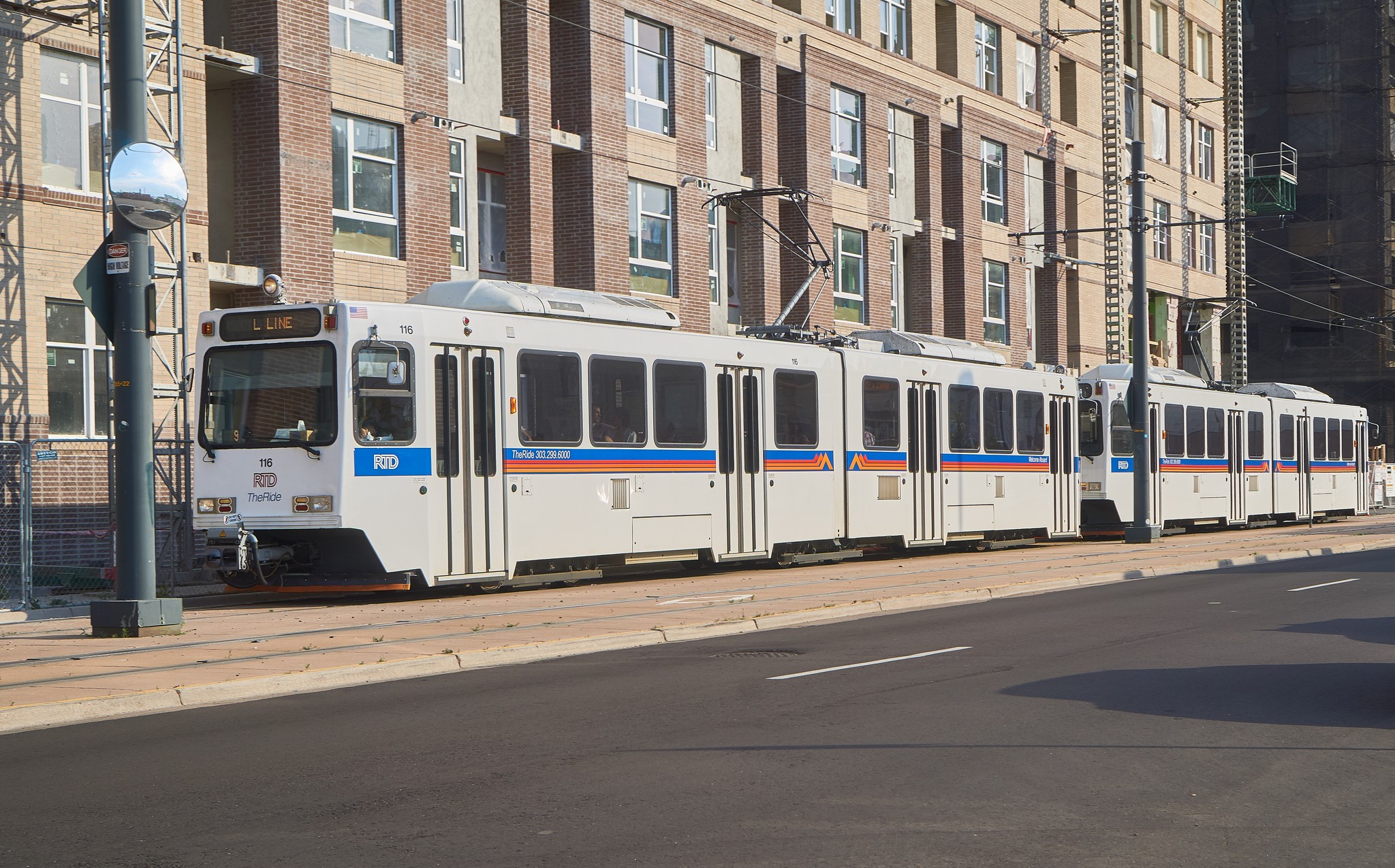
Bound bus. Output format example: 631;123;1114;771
198;186;1383;603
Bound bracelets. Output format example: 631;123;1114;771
363;427;366;431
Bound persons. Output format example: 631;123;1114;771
592;404;615;440
601;407;636;442
358;416;394;441
865;428;875;446
